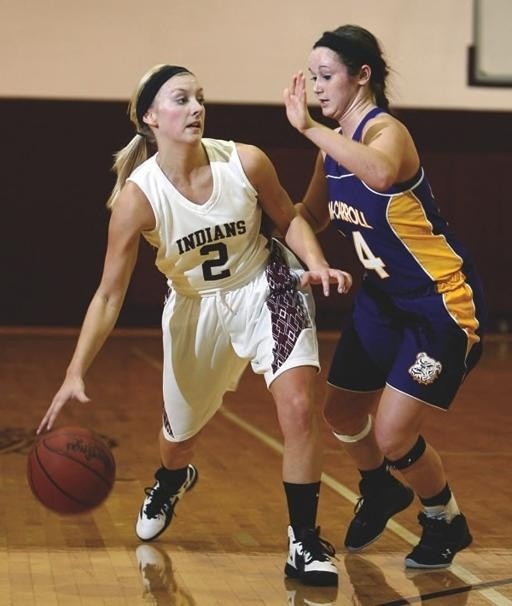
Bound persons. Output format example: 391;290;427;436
285;22;484;569
34;64;352;589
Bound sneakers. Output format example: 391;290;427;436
285;524;340;586
135;464;197;541
344;473;414;551
405;512;473;570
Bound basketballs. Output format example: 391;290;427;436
28;427;115;514
